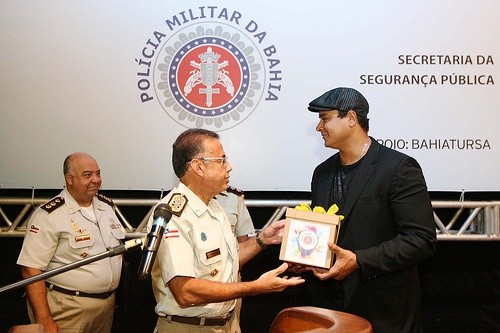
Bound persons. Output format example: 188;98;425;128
285;87;437;333
212;185;255;323
146;128;305;333
16;152;127;333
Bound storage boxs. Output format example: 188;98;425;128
279;207;341;269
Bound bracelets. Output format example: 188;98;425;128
255;233;268;249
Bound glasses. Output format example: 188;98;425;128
187;155;228;165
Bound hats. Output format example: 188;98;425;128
307;86;369;116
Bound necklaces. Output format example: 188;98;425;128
360;136;371;158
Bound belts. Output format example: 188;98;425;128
45;281;115;299
165;314;231;326
